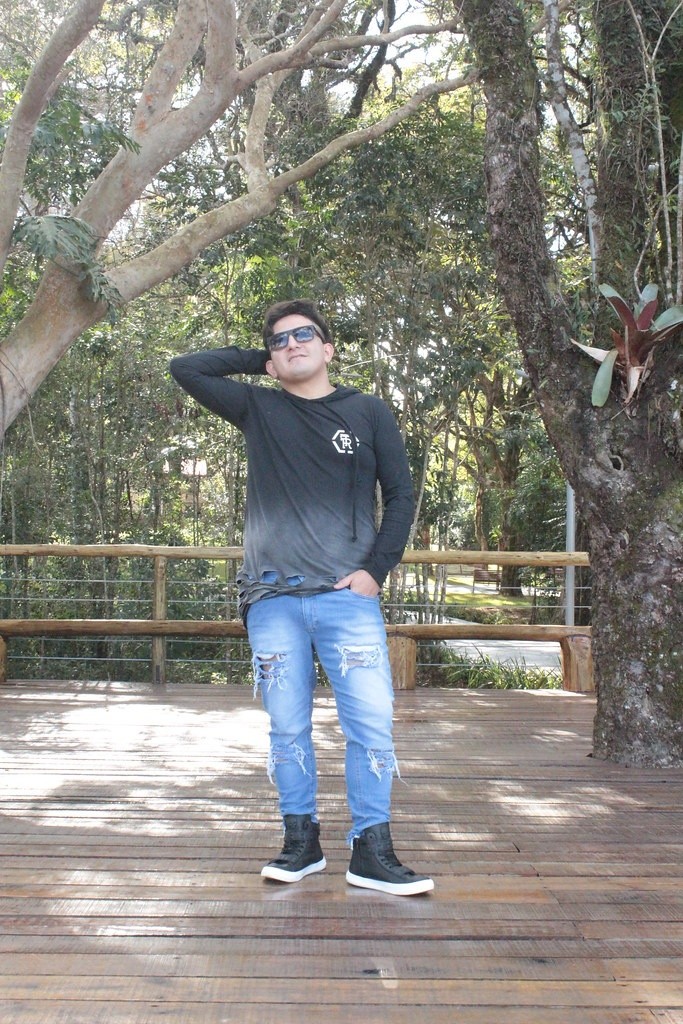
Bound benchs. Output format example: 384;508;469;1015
519;567;565;595
473;569;502;595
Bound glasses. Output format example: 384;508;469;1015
267;324;325;351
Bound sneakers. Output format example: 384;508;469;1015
345;822;434;896
260;814;327;883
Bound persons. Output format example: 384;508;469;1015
166;298;436;895
